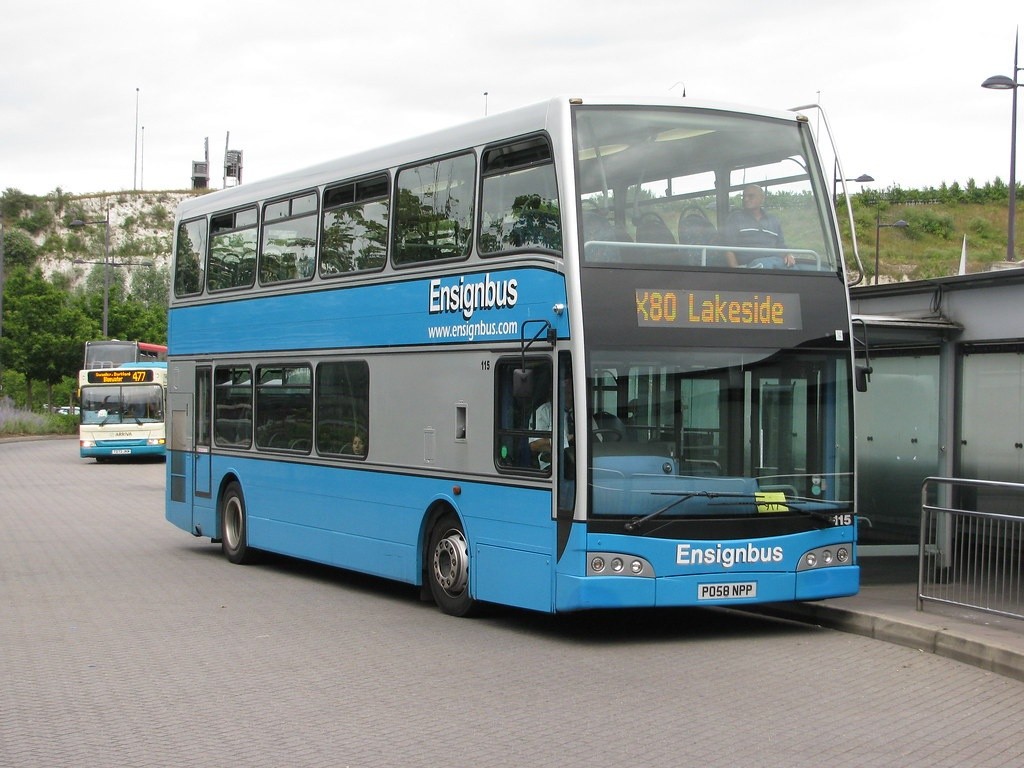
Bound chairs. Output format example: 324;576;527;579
580;198;721;246
214;400;354;453
596;411;627;441
210;226;441;291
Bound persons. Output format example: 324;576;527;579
723;183;795;268
352;432;367;455
528;375;605;473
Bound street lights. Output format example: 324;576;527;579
69;199;109;340
833;174;874;208
874;220;908;284
981;74;1024;260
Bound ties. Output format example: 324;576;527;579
565;408;575;437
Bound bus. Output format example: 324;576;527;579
84;339;168;369
164;97;872;617
77;362;167;462
